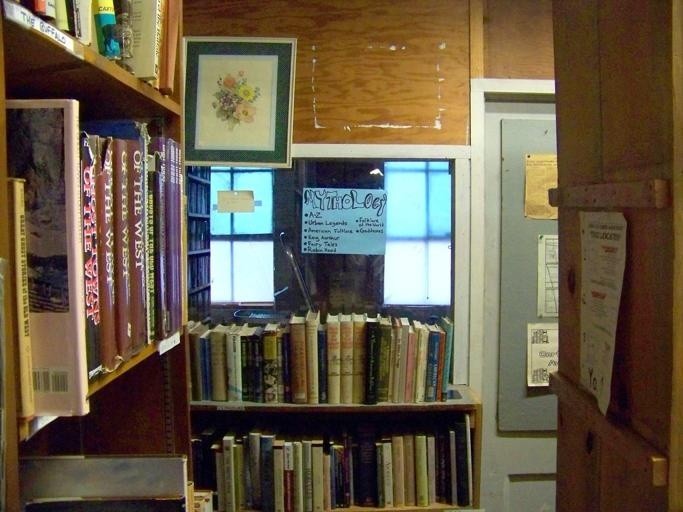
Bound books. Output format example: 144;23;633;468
20;0;179;95
4;99;181;415
216;414;472;512
186;166;211;320
188;312;453;404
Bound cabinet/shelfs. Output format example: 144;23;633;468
191;386;483;510
1;0;193;512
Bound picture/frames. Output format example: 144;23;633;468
183;36;298;169
